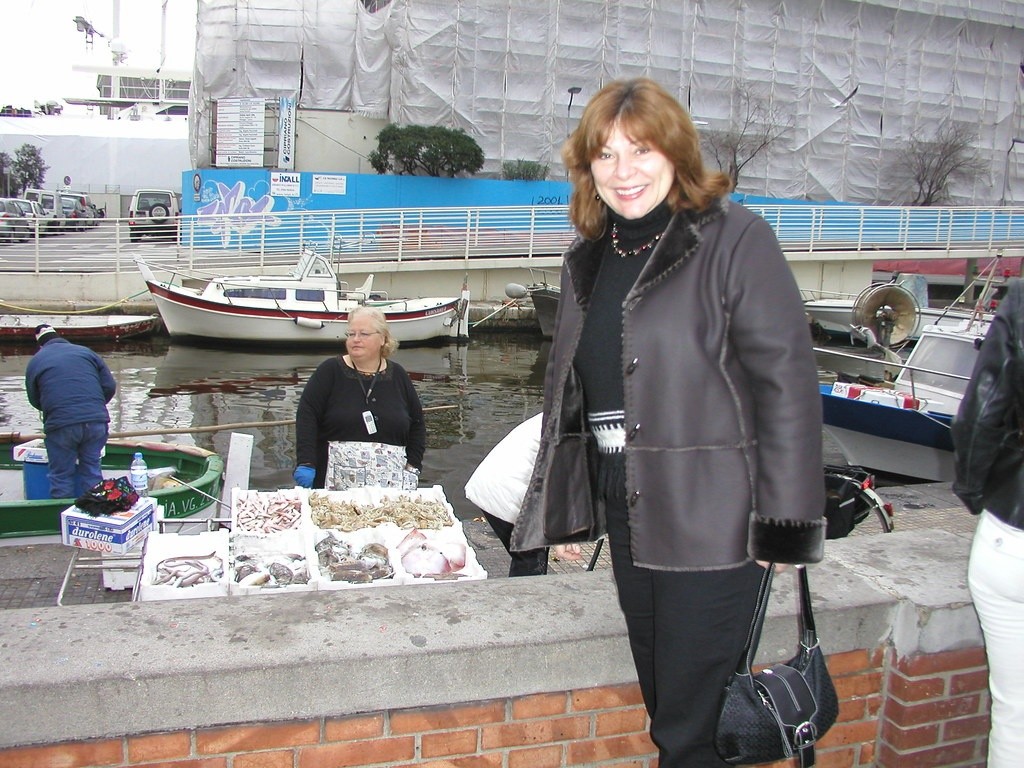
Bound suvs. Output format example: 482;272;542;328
126;189;183;242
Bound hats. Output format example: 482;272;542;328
35;324;60;346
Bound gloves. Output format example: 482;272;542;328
291;463;316;489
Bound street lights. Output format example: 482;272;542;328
1000;137;1024;206
565;86;582;181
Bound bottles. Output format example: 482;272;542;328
131;453;148;497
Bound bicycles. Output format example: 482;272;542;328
822;461;896;541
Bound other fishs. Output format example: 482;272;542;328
151;551;223;588
235;492;301;534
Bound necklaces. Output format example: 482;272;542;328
612;223;665;258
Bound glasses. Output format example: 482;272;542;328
345;331;380;339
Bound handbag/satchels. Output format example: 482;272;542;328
714;562;838;765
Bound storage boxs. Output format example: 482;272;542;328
13;439;106;464
101;541;144;591
22;462;51;500
60;500;153;556
831;381;919;410
140;484;488;602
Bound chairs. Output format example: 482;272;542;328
140;199;150;208
346;274;374;305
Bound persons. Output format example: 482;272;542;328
292;307;425;489
952;277;1024;768
465;411;549;577
26;324;116;498
509;78;828;768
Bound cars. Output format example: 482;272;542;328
0;188;105;244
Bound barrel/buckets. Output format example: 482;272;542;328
23;461;84;500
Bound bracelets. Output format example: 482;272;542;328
409;467;420;476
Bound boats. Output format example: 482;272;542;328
815;251;1013;483
527;266;563;337
130;240;472;345
799;271;998;342
0;309;160;343
0;429;225;545
